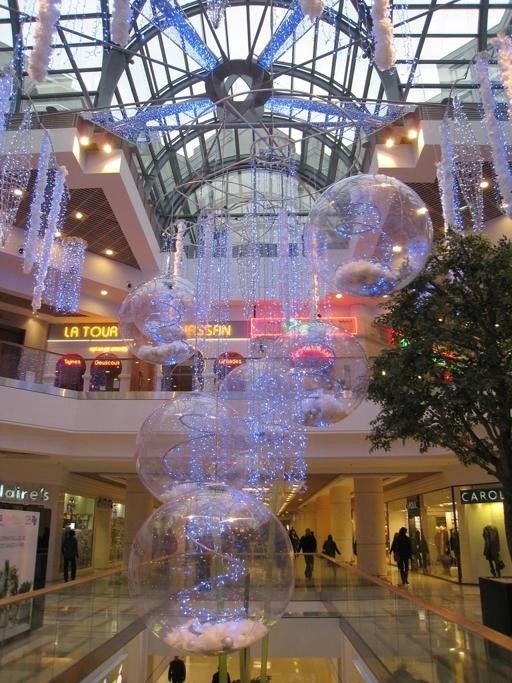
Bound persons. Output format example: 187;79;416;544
212;664;231;683
61;529;80;583
298;528;317;579
321;533;340;563
389;532;401;569
167;654;187;683
392;526;412;584
310;531;314;536
289;529;299;560
482;524;501;577
433;522;460;575
